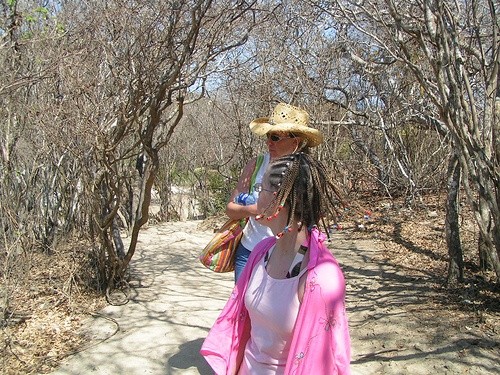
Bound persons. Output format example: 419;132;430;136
225;102;323;287
199;152;350;375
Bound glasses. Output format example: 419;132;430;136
267;133;291;141
253;183;273;193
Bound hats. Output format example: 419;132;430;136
249;103;323;147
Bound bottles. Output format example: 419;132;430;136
235;192;255;204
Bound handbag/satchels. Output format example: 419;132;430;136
200;154;264;273
